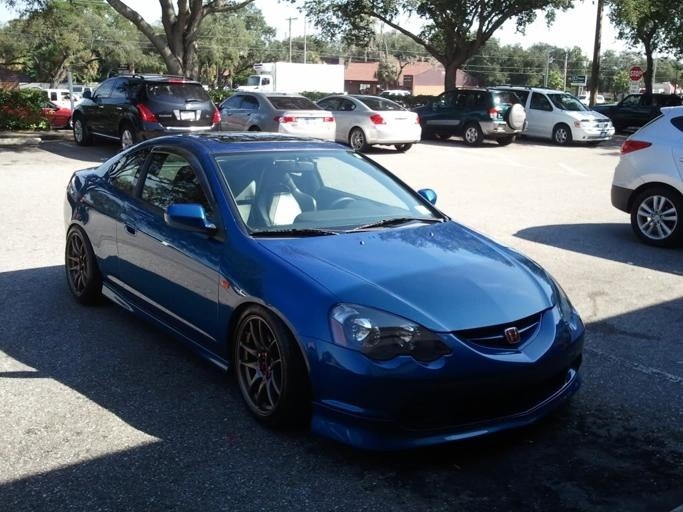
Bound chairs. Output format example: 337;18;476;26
248;163;316;228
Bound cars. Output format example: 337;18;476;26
56;127;592;457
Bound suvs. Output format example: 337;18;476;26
607;104;683;246
